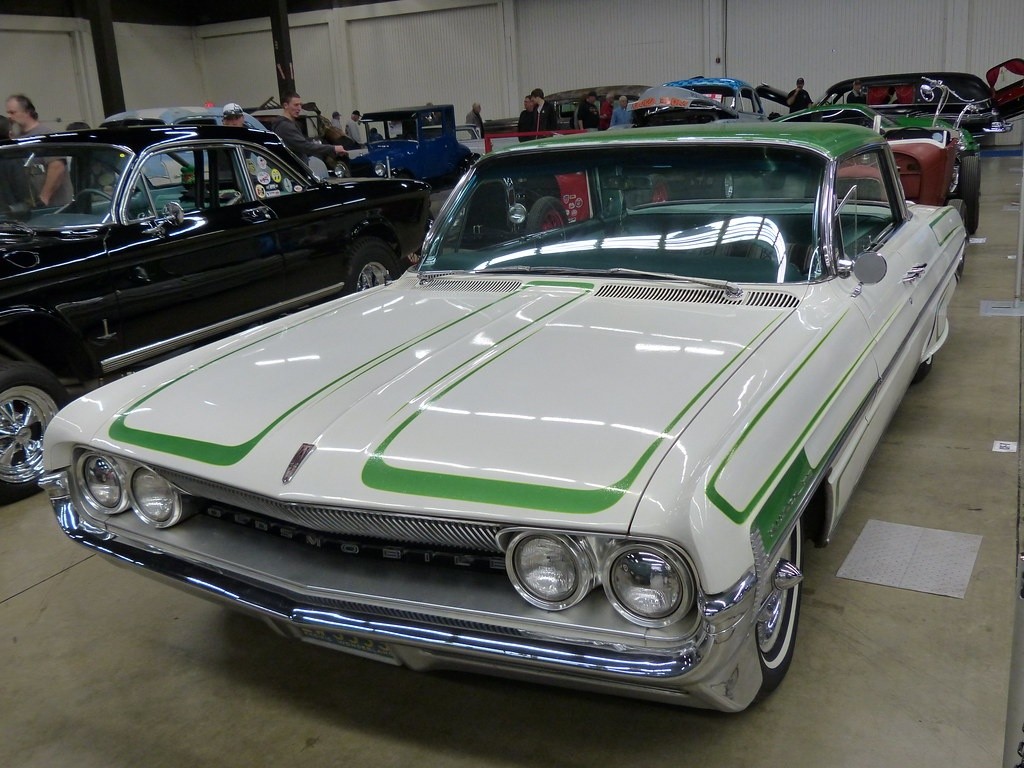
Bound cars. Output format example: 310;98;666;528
834;126;980;235
482;86;740;134
422;124;565;162
42;122;968;714
769;104;980;149
0;127;432;507
661;76;771;122
334;104;474;194
755;57;1024;139
100;106;270;186
249;108;370;179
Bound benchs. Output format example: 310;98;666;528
614;203;892;256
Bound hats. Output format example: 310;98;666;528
370;128;377;133
223;103;243;119
588;91;597;96
853;80;861;85
797;78;804;84
332;112;341;116
352;110;362;117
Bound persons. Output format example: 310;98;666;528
577;91;632;131
331;110;362;144
846;79;867;104
784;77;813;114
369;127;384;141
0;95;117;223
518;88;556;143
221;89;349;179
465;102;485;140
